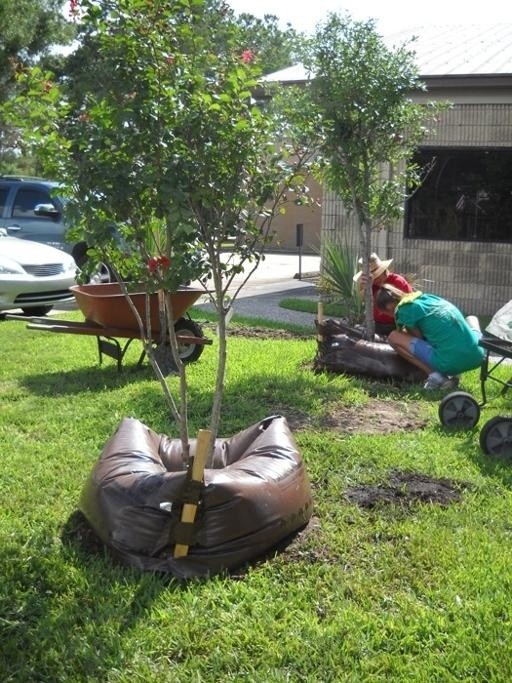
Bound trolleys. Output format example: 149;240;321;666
439;337;512;458
6;282;216;373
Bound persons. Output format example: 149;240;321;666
352;254;412;332
375;283;485;391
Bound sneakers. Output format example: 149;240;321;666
423;373;460;392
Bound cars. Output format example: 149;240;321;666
0;174;131;321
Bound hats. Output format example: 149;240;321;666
352;252;393;284
